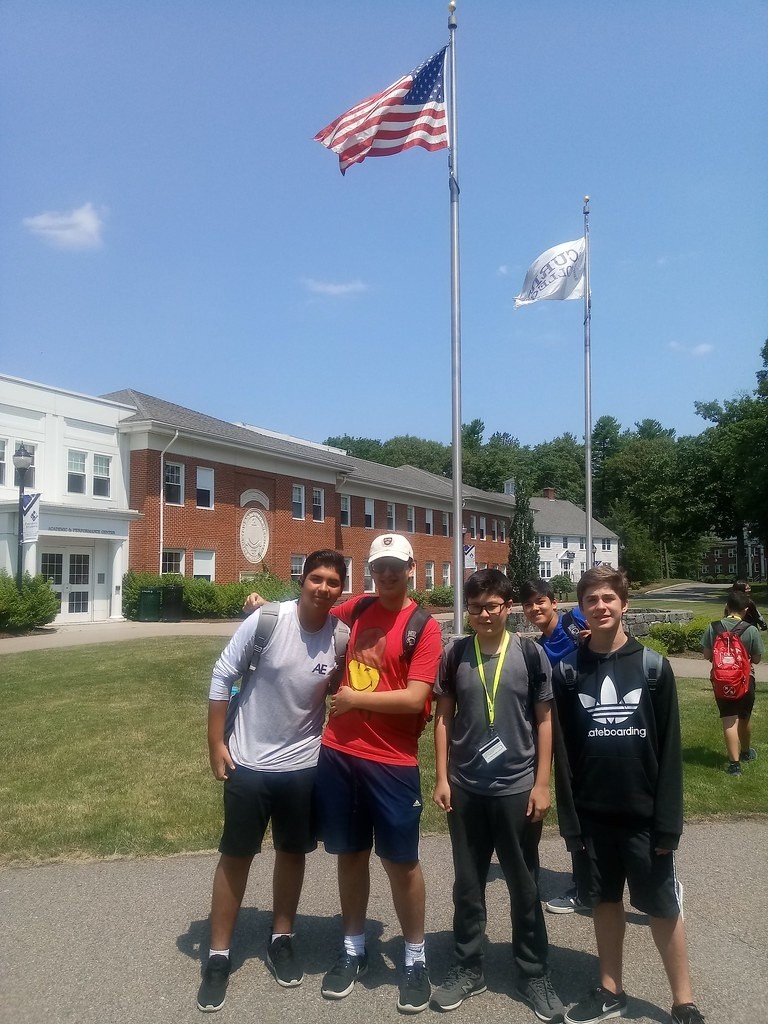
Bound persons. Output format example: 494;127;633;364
552;565;705;1024
196;548;349;1010
431;569;566;1024
243;532;442;1011
700;591;765;777
723;579;768;631
519;578;684;921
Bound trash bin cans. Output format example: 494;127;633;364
139;584;185;622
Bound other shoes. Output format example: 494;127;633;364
728;764;742;776
740;748;757;762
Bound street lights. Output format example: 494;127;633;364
461;524;467;606
13;440;34;593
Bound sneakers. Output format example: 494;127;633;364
564;987;627;1024
266;933;304;987
197;955;231;1012
515;971;564;1024
671;1003;704;1024
322;947;369;998
546;896;591;914
430;966;487;1011
398;961;432;1012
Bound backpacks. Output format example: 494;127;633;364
222;601;349;748
712;620;751;700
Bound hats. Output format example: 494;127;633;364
368;534;414;563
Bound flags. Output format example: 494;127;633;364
514;237;586;310
313;45;449;174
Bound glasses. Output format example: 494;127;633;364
466;601;506;615
371;562;404;573
745;587;751;591
